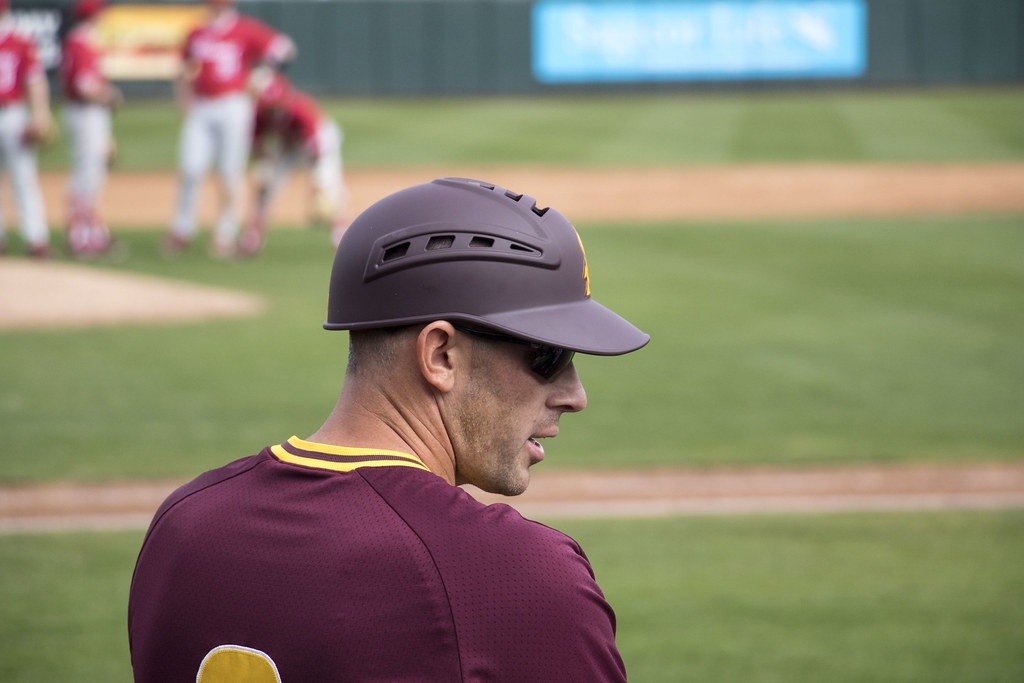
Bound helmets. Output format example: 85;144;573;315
323;179;651;356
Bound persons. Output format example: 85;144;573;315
160;1;348;257
0;0;124;259
127;178;650;683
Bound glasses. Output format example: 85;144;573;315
455;325;575;383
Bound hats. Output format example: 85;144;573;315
78;1;102;18
